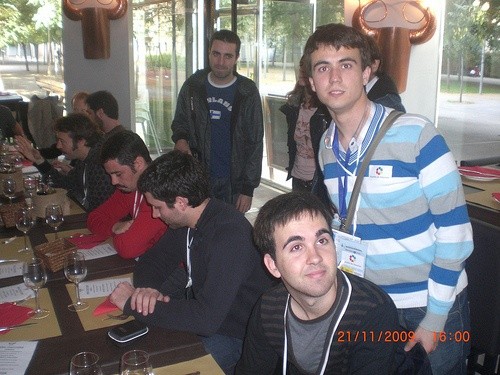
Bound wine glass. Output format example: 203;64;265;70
45;203;65;239
23;176;40;211
14;208;34;254
21;258;50;319
2;179;17;207
63;252;89;311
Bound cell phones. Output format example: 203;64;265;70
107;319;148;342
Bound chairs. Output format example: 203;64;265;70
18;94;64;159
135;101;161;157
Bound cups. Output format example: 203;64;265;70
69;352;104;375
120;349;152;375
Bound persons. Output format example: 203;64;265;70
87;131;168;260
171;30;264;213
235;191;399;375
300;24;475;375
364;35;405;113
279;55;331;191
108;150;275;375
0;90;153;214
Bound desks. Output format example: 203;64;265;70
463;159;500;375
0;138;230;375
0;91;23;121
